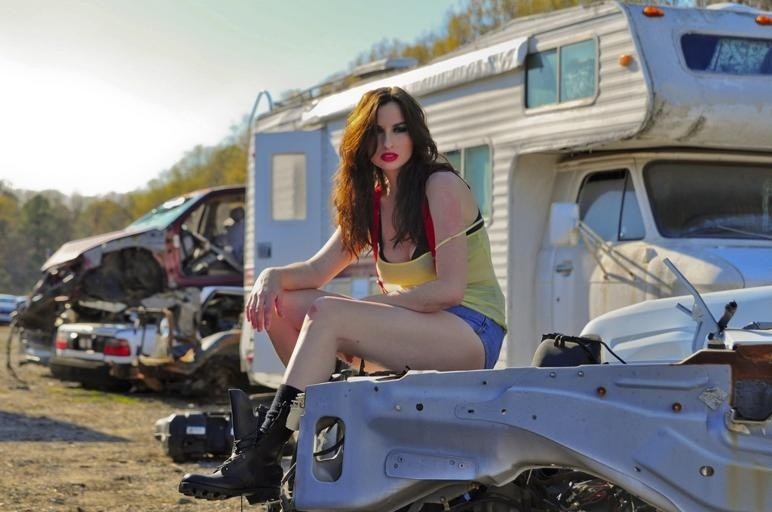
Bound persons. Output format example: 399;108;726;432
214;206;245;266
177;85;507;506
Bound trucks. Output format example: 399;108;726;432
239;0;772;390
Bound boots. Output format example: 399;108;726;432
178;388;291;506
